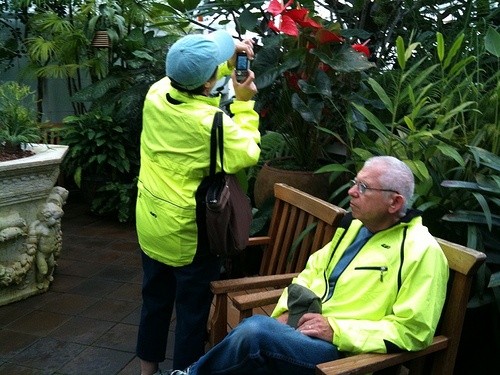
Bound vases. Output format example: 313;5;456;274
79;171;130;218
253;156;339;255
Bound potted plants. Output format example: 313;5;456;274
0;80;70;308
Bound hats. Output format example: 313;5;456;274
166;29;235;91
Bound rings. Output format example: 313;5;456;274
309;325;312;329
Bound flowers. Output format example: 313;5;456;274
248;0;379;167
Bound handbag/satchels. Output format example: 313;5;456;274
195;112;252;253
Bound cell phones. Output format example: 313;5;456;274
236;52;252;81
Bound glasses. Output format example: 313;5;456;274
348;180;400;195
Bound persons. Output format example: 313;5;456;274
154;155;449;375
134;31;263;374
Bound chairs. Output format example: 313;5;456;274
205;183;487;375
